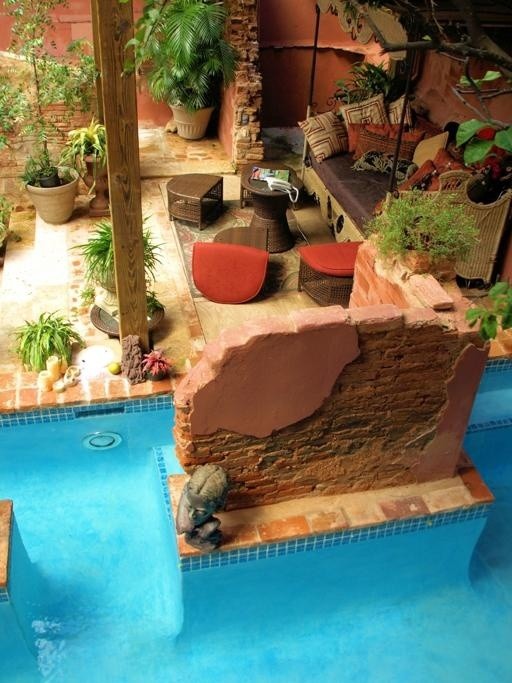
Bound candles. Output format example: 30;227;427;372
38;356;65;393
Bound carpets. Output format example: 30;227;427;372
157;172;319;341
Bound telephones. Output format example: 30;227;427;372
266;176;292;192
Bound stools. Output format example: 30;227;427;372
238;160;297;210
166;172;225;230
190;226;269;304
297;239;366;309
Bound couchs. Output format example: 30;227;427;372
296;104;511;288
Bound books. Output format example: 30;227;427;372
250;164;292;185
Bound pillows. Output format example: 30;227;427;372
298;92;469;191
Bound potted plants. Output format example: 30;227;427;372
364;186;479;274
1;197;13;250
137;0;242;139
75;205;163;305
142;344;174;384
0;0;112;225
